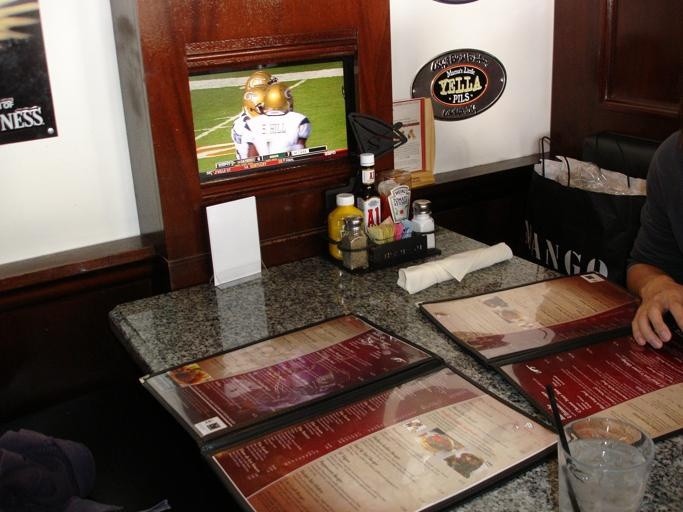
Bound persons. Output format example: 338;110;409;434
230;85;267;160
626;129;680;347
244;81;312;164
232;71;278;126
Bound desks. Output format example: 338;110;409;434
102;213;679;510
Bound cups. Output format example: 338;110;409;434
557;416;655;512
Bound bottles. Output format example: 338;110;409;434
328;152;436;270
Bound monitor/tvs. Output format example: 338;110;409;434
187;55;355;189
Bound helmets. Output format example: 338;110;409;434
242;70;294;119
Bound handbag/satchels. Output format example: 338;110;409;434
522;132;647;290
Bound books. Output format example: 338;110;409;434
416;267;681;455
132;310;560;512
